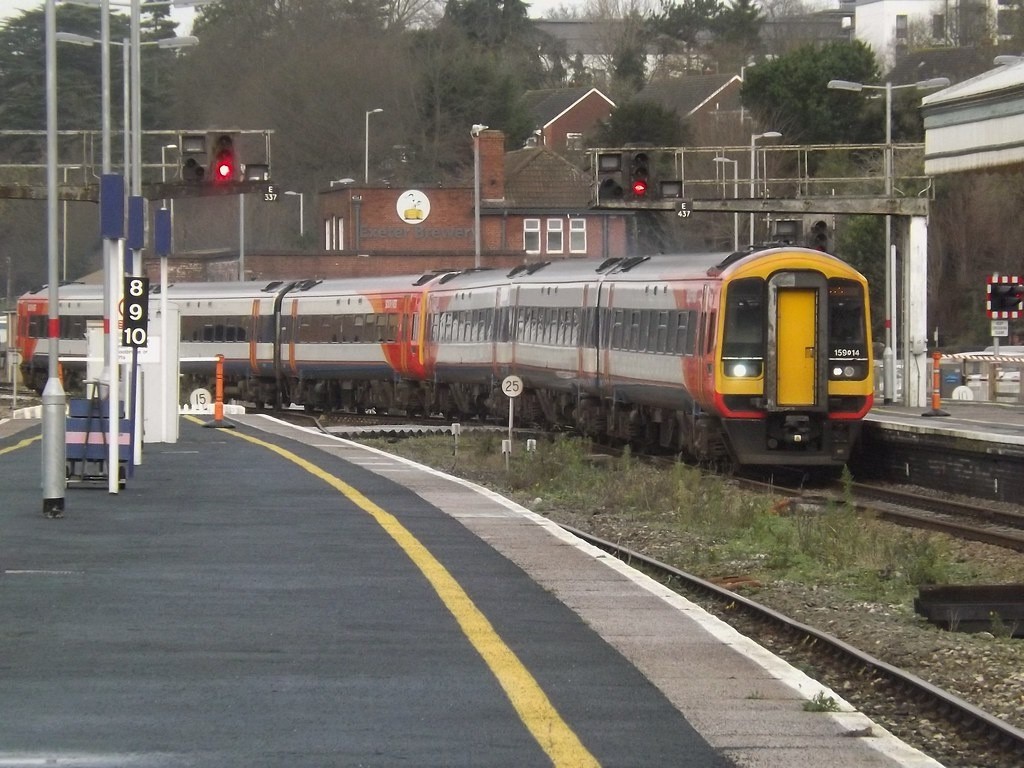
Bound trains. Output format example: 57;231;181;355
12;240;876;486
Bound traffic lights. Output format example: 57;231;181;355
630;149;651;201
209;132;236;182
809;220;829;256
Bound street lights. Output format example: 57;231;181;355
365;108;384;184
470;122;490;269
160;145;179;198
712;157;739;252
54;30;202;457
749;132;783;252
284;190;304;238
54;0;212;465
826;77;953;404
